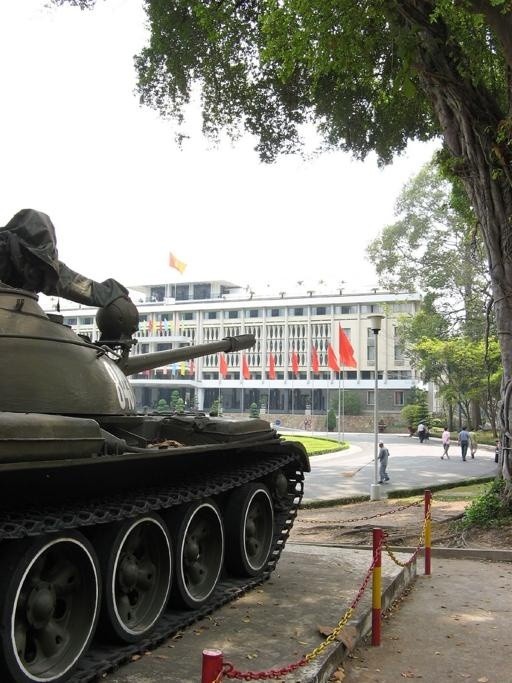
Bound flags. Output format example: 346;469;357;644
338;322;357;368
243;352;251;378
269;350;277;379
141;357;196;377
168;253;187;274
327;342;341;373
138;317;185;333
219;352;228;378
312;345;319;375
292;350;299;376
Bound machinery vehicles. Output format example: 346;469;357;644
0;209;310;678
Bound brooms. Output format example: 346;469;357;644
342;457;383;477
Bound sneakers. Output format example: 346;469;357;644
379;477;389;483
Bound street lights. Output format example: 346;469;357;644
366;313;385;500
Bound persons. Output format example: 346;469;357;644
308;419;313;429
458;424;469;461
417;420;426;444
493;439;501;463
407;423;414;439
303;417;308;429
440;426;451;459
377;440;390;485
469;427;478;459
